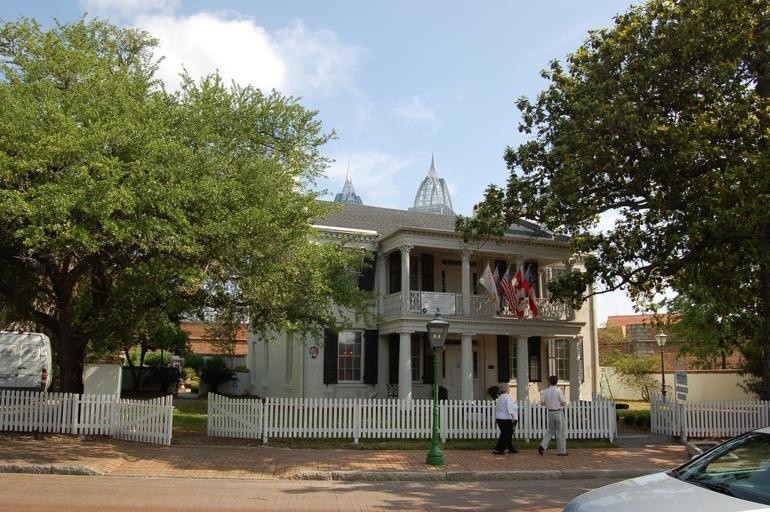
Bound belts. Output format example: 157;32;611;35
549;409;560;411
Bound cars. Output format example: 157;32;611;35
560;422;769;511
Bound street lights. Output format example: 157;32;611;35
425;306;450;466
653;328;669;405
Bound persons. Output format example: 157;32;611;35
492;383;518;454
538;375;570;455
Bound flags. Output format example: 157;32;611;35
479;264;538;320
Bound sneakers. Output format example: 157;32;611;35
493;450;518;455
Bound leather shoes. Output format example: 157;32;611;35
557;454;569;456
538;445;544;456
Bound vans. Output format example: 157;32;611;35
1;330;54;394
